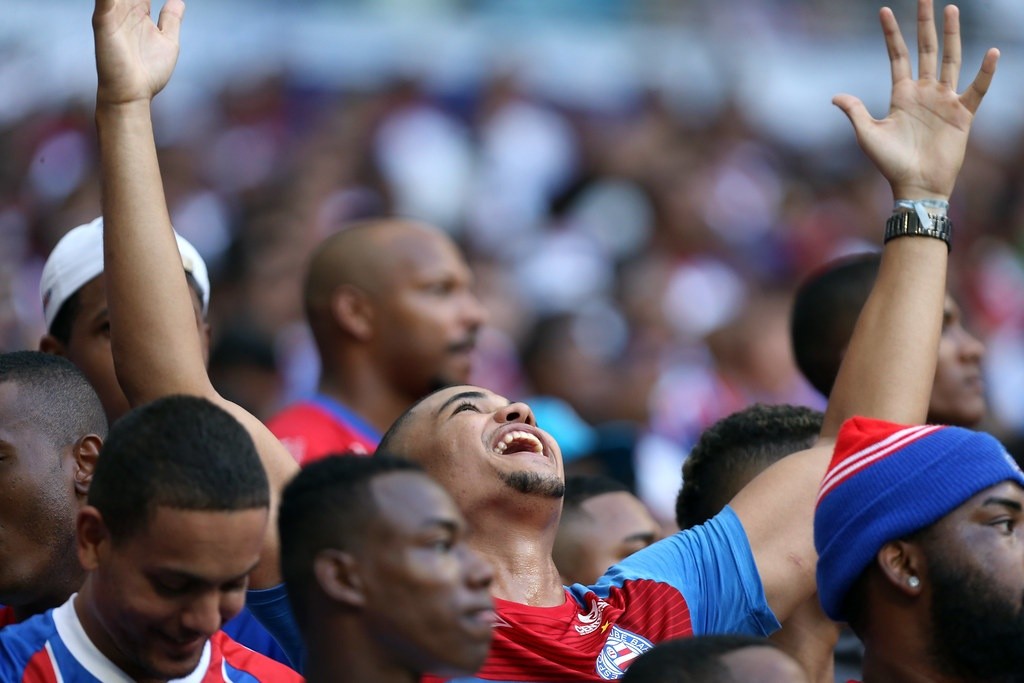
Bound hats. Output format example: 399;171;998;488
812;413;1023;627
39;214;212;335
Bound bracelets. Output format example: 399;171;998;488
892;197;950;229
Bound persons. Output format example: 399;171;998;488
0;0;1024;683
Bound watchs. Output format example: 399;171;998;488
884;211;953;255
